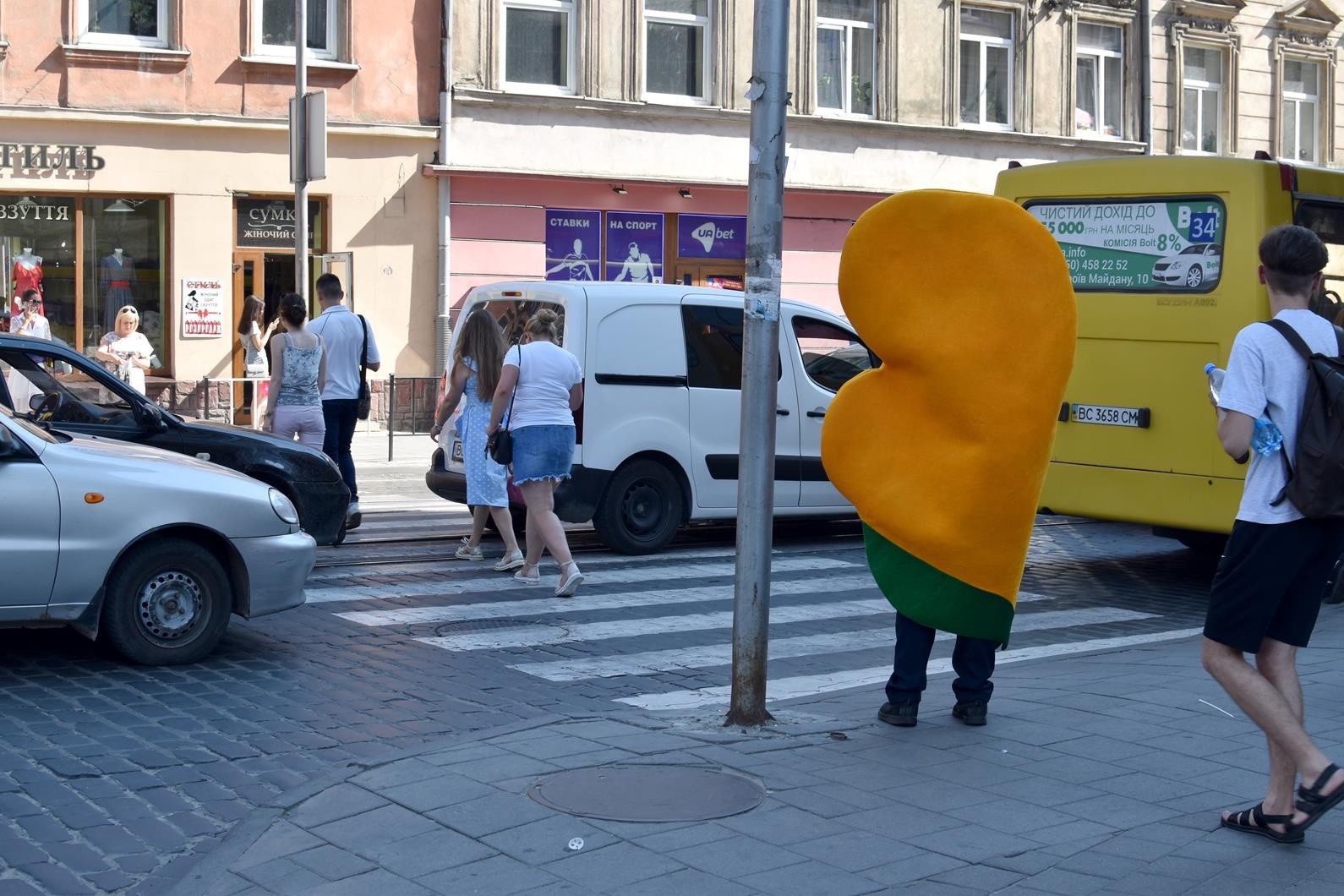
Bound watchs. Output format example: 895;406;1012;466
435;424;443;431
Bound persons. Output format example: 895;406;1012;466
429;307;584;597
306;274;381;546
262;293;326;450
545;240;594;282
237;295;280;427
95;305;154;404
11;248;44;318
7;290;52;414
100;248;136;335
1201;225;1344;843
612;241;654;283
878;612;996;727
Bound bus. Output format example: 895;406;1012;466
995;150;1344;606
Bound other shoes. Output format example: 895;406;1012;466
344;503;362;530
952;699;988;724
878;699;919;724
357;381;372;420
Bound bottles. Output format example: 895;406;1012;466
1204;364;1282;457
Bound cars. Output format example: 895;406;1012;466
0;332;351;545
0;404;317;665
804;343;882;392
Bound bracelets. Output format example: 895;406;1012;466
263;407;274;419
19;326;26;330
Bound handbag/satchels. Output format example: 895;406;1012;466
484;425;514;475
103;361;131;406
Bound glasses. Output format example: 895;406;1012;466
28;299;42;305
122;308;136;313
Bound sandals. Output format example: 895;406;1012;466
1220;801;1306;844
1283;763;1344;841
513;561;541;585
554;560;584;595
455;537;484;561
493;549;525;571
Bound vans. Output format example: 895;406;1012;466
425;281;881;555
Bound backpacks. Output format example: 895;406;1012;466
1258;318;1344;541
